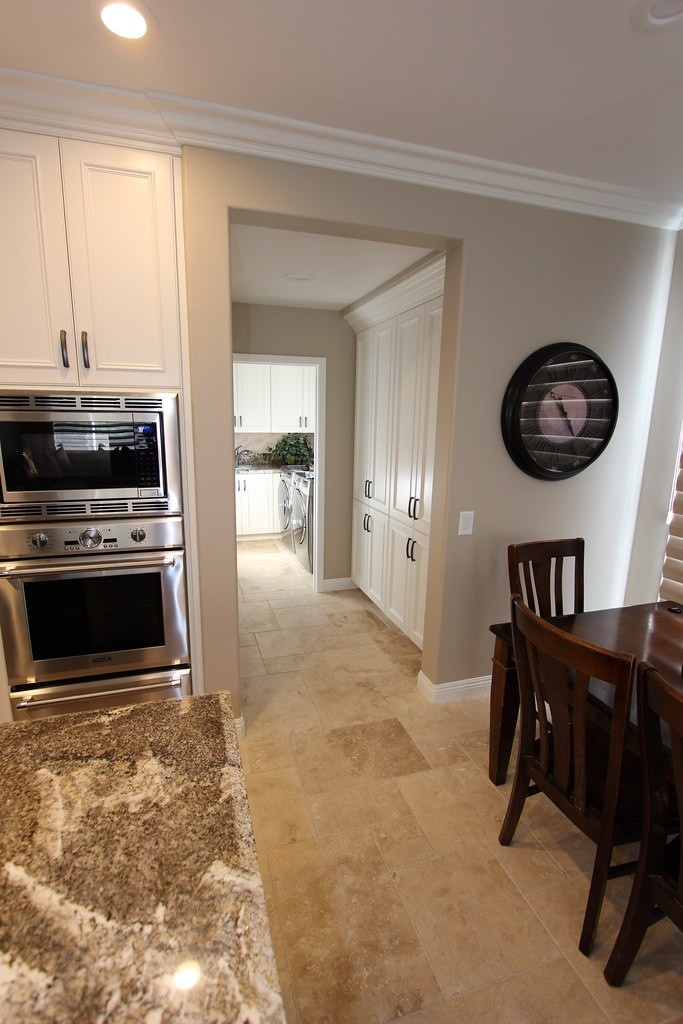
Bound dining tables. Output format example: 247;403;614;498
489;600;683;782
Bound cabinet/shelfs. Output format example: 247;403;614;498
235;469;282;541
232;363;314;433
350;294;443;650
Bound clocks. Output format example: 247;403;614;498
501;342;619;481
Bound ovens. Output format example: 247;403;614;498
0;388;193;723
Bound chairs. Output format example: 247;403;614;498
603;661;683;987
508;538;584;617
499;593;680;957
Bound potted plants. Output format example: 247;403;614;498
265;434;314;465
